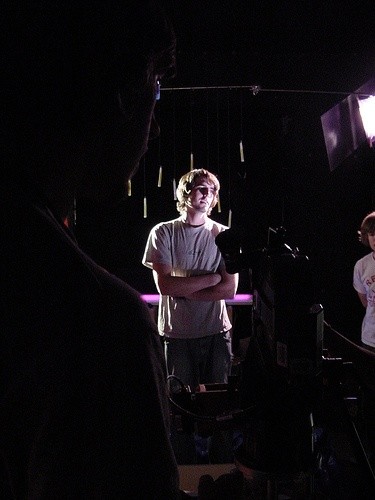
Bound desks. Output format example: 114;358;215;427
140;294;253;327
